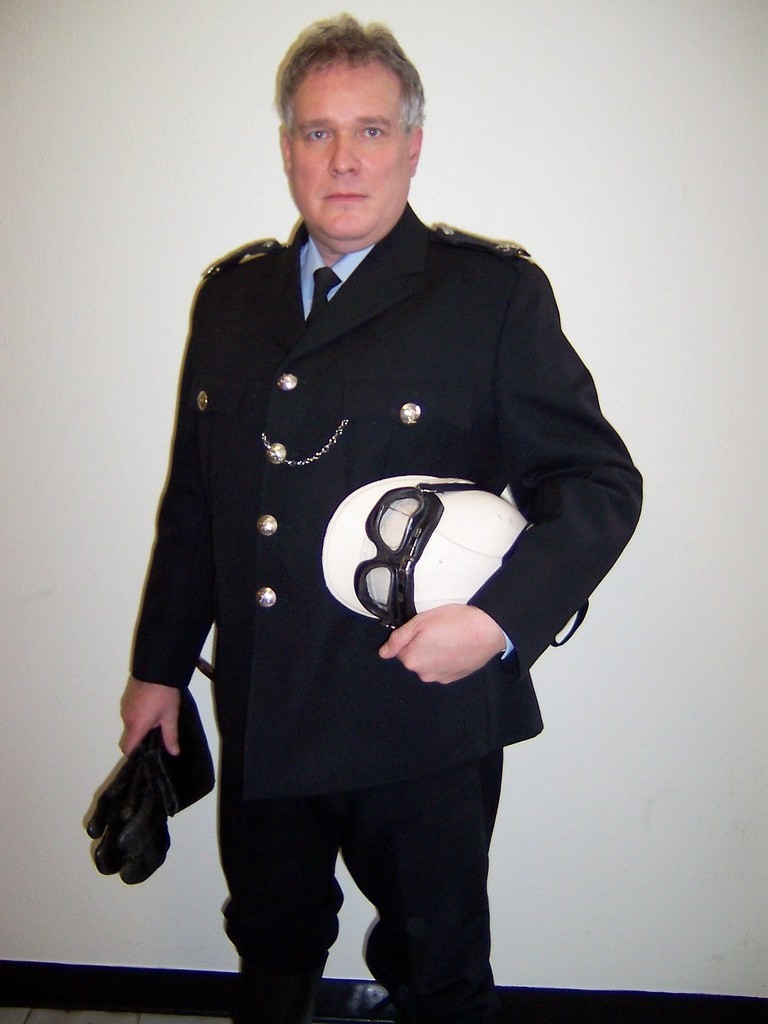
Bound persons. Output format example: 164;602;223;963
120;17;643;1023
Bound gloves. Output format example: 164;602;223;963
87;687;215;885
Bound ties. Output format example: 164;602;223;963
306;267;342;328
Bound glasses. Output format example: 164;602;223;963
353;483;490;629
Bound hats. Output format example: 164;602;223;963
321;475;529;621
